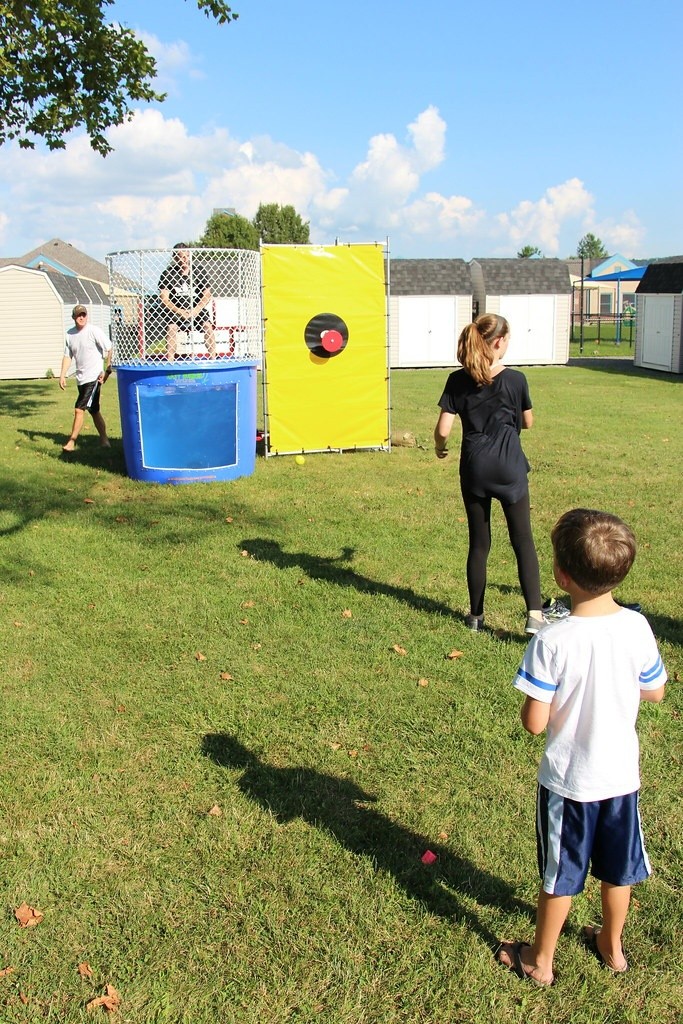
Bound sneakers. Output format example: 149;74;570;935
465;613;485;631
524;615;551;636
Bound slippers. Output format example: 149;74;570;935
497;941;555;987
584;923;628;972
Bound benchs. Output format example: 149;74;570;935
137;297;258;360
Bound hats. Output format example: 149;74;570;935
72;305;87;316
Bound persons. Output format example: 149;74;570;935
497;508;669;988
157;243;216;362
59;304;113;452
435;313;552;634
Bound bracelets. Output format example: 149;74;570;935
106;370;111;374
437;448;443;451
